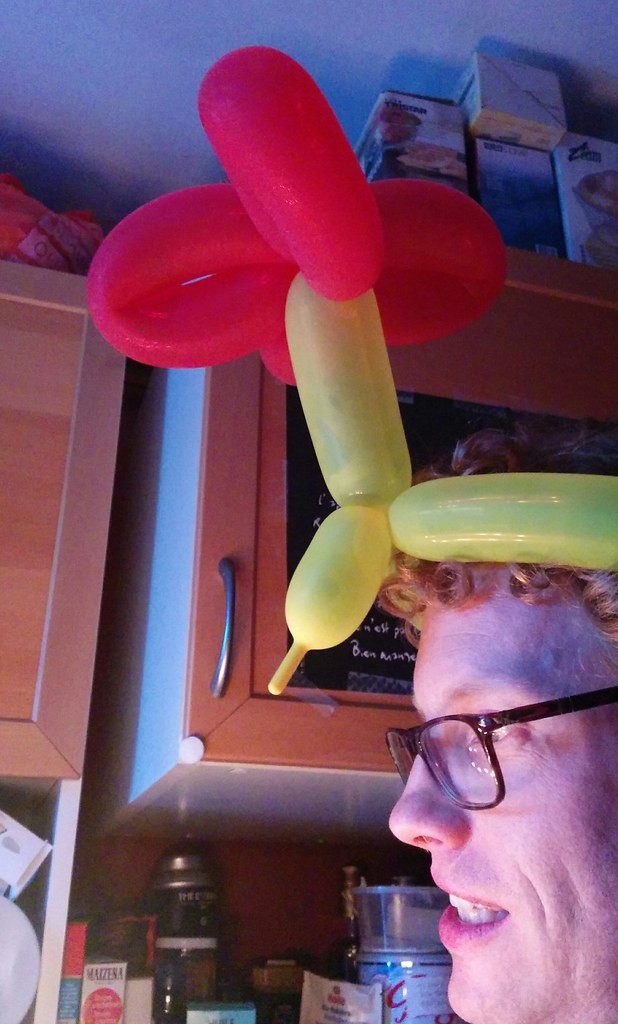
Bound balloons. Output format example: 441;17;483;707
83;46;618;695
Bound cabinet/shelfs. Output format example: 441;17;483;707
77;255;616;851
2;268;127;786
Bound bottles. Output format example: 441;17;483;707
330;861;364;985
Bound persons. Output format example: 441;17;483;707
359;432;618;1023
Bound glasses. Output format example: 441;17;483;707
381;684;618;810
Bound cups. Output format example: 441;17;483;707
151;848;223;1024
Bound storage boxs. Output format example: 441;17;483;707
343;50;618;268
